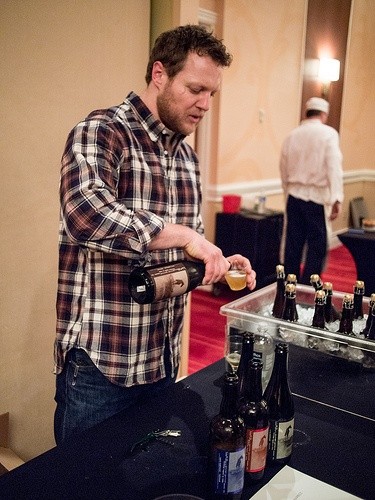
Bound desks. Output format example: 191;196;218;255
338;225;375;298
1;348;375;500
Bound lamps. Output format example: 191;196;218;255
318;58;341;100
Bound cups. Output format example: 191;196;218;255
224;261;246;290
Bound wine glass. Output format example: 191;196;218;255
224;335;242;373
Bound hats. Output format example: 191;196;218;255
305;97;330;113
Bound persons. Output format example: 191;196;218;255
51;26;257;447
276;96;344;288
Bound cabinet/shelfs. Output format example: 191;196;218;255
209;207;284;298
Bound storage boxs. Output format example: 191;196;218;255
220;278;374;431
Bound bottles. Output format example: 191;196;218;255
333;296;358;353
277;273;298;317
307;290;330;349
256;188;266;213
262;344;294;463
322;281;333;304
235;333;254;407
272;265;287;317
208;372;245;500
236;358;270;482
254;323;275;395
353;281;365;320
310;273;341;322
282;283;300;322
359;294;375;368
129;260;205;305
